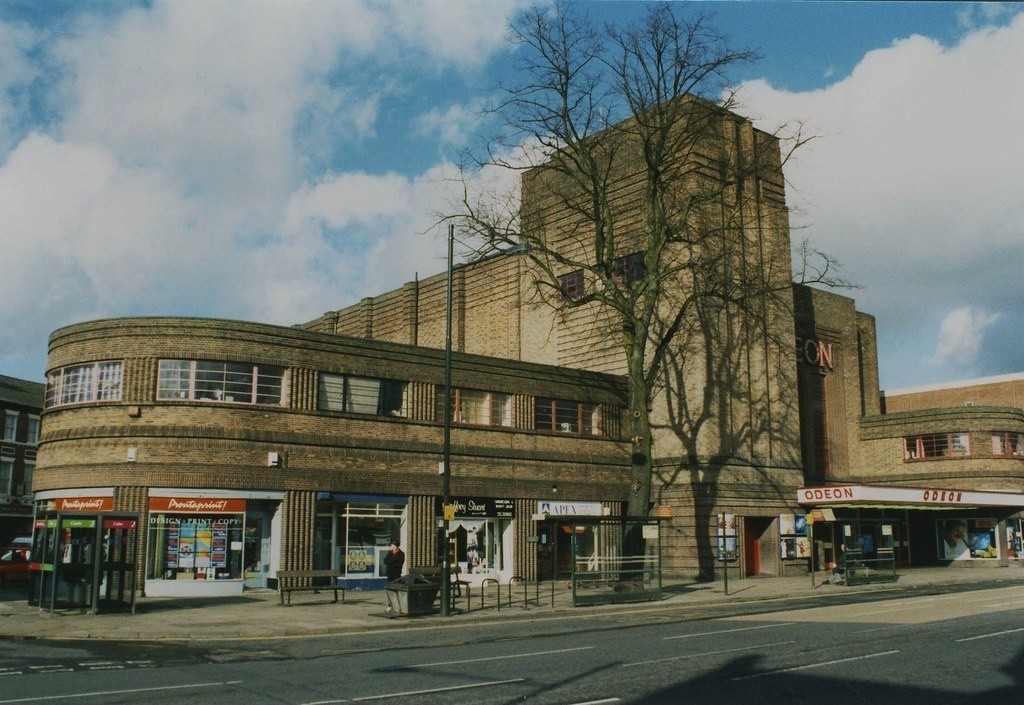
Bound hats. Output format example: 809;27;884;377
389;541;398;547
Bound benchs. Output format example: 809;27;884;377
409;567;471;600
276;571;346;606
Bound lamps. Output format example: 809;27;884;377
268;450;278;466
128;405;140;417
438;461;445;475
126;447;137;463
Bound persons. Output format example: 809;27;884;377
949;521;971;548
384;540;405;611
860;527;873;568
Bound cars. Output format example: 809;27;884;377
8;537;33;548
1;550;30;561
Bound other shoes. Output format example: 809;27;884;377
384;607;392;612
822;580;830;585
836;581;845;586
